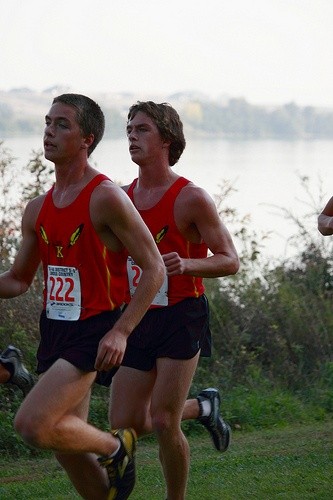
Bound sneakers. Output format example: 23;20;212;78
96;426;138;500
197;388;231;452
0;344;34;397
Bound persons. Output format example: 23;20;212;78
0;345;34;396
317;194;333;236
108;100;240;500
0;94;166;500
